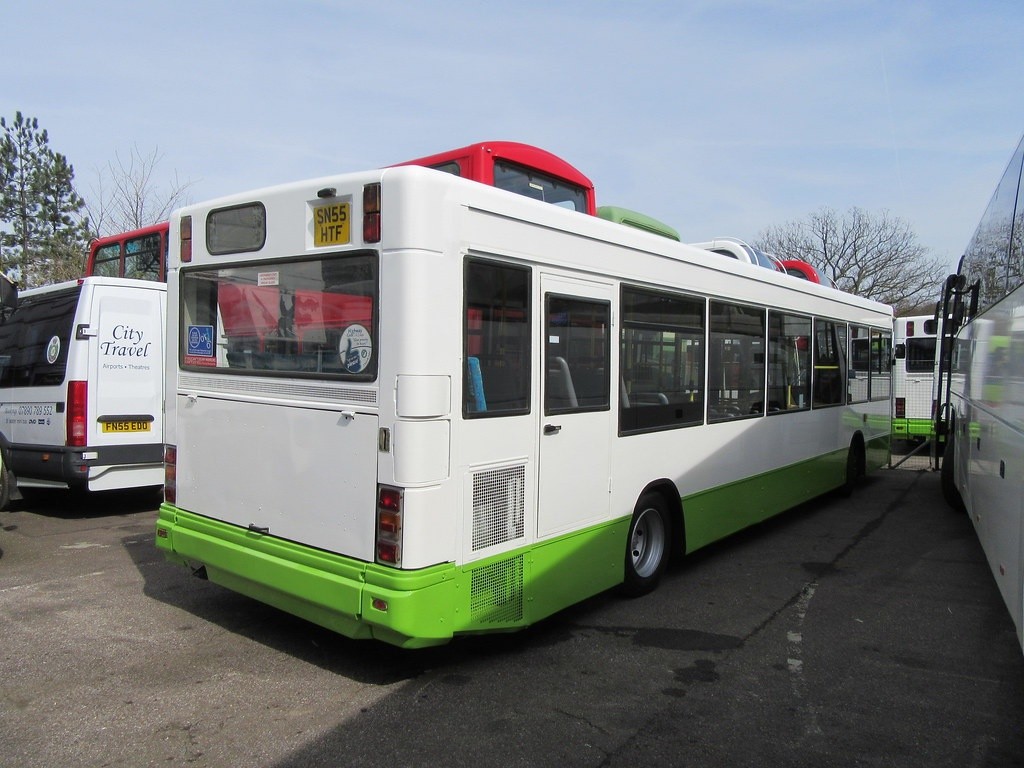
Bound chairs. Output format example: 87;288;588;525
544;355;579;412
300;327;350;373
592;365;630;412
469;352;523;414
638;384;792;428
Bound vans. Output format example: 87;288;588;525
0;275;232;515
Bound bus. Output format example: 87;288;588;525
592;207;686;397
841;313;949;445
929;135;1024;655
83;139;601;373
946;312;1023;458
157;164;907;660
772;260;843;380
683;234;789;406
